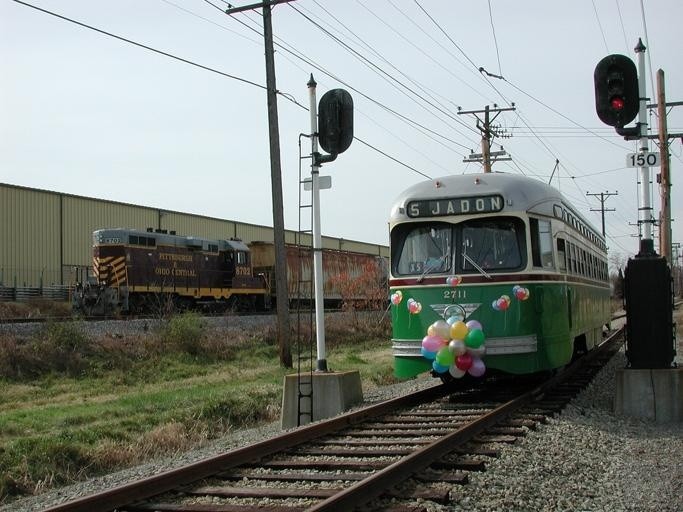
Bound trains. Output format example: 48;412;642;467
389;171;611;379
71;228;390;318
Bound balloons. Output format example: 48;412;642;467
419;316;486;379
491;285;530;312
389;290;422;314
445;273;462;288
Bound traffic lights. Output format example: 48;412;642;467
595;54;640;129
